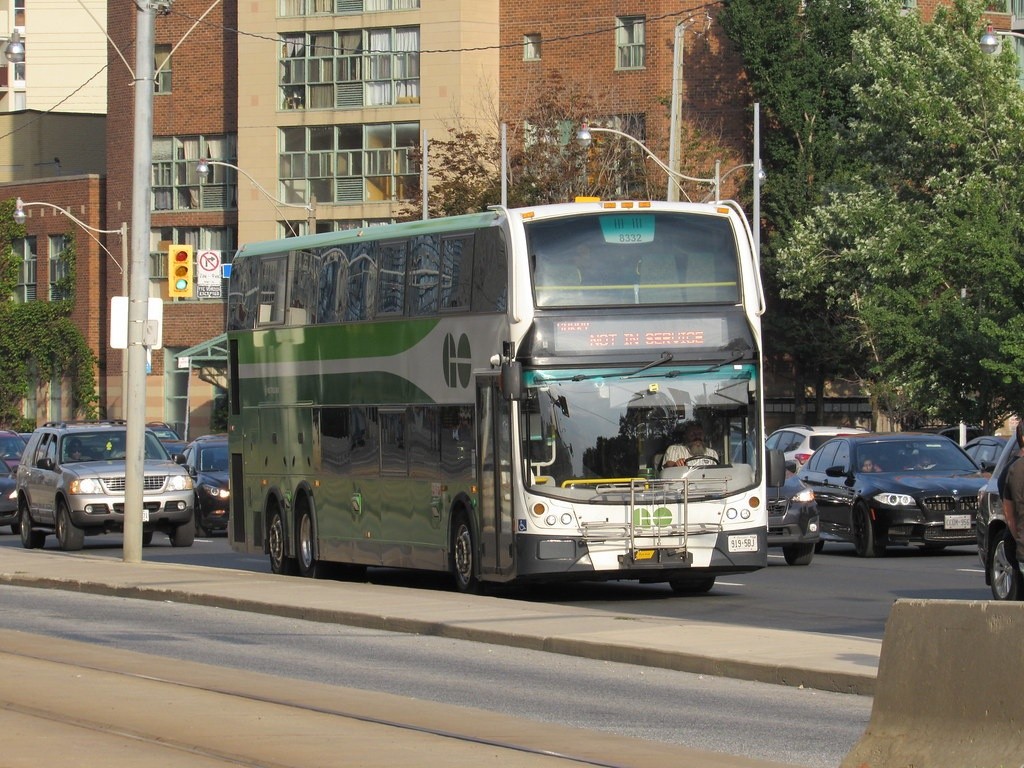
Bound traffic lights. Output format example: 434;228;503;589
167;244;197;300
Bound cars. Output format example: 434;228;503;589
728;442;821;566
917;426;984;457
180;433;230;537
974;416;1024;601
960;435;1021;475
793;432;997;557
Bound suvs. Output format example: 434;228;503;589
0;429;34;534
16;419;197;552
147;421;189;461
766;424;874;479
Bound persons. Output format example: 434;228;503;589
997;419;1024;574
857;455;880;472
915;451;935;470
66;440;93;462
661;421;719;469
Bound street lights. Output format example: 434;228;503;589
13;196;131;422
196;156;319;233
575;122;767;203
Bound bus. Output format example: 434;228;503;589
219;195;769;598
